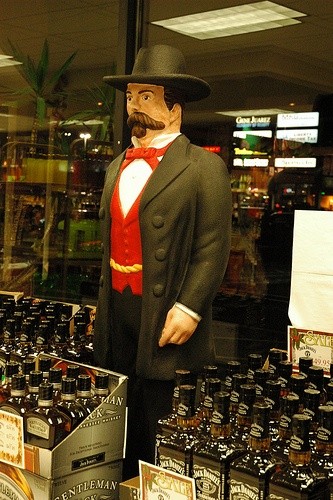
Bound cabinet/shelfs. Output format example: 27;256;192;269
1;139;113;303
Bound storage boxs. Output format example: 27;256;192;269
0;291;140;500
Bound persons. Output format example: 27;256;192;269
86;40;231;477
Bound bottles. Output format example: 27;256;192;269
153;348;333;500
0;293;109;449
